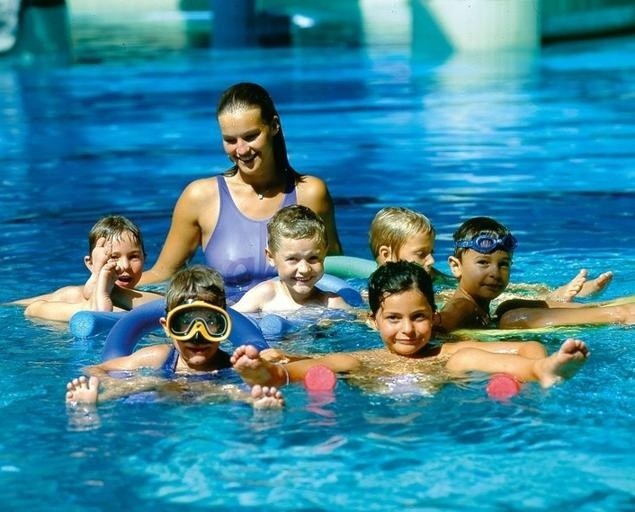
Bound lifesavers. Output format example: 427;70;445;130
99;298;271;408
320;257;378;281
240;271;364;336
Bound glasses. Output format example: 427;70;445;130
165;298;232;344
452;232;517;255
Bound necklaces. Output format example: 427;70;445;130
233;169;275;200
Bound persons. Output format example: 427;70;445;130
64;264;287;411
230;203;350;325
1;213;165;322
369;206;614;305
229;259;593;391
437;217;635;326
135;83;345;305
